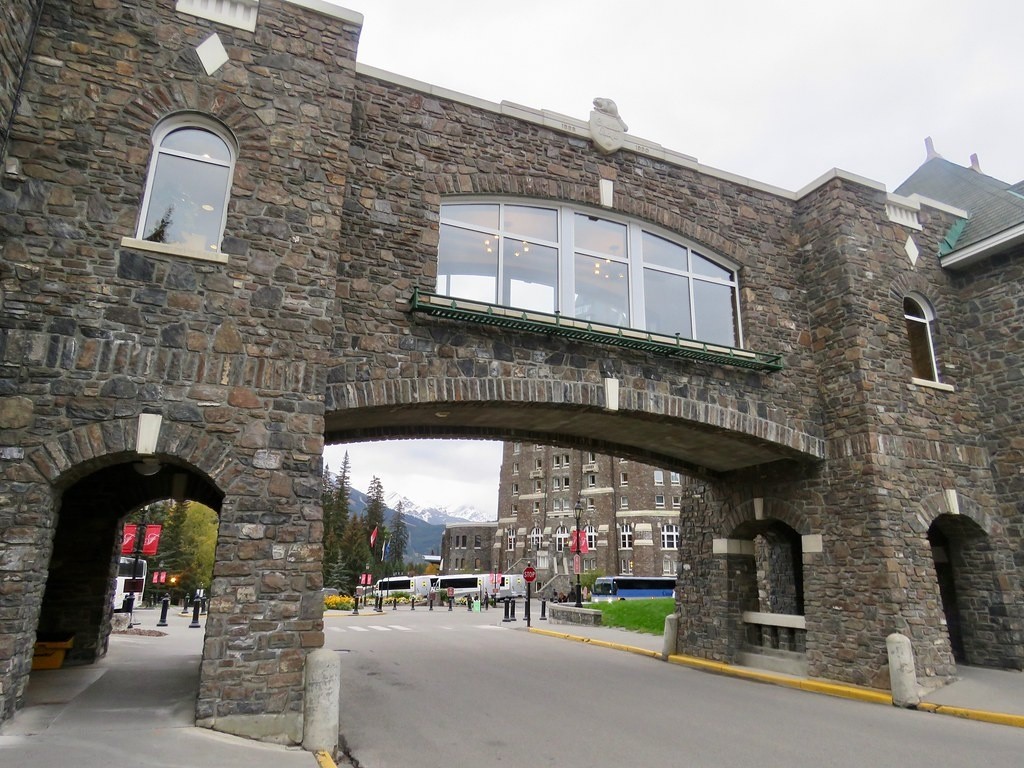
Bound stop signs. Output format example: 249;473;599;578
523;567;537;581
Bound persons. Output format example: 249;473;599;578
553;587;576;603
464;592;489;611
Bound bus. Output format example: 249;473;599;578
591;576;677;603
114;557;147;610
354;573;525;602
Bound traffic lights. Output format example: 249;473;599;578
629;559;633;568
623;559;626;570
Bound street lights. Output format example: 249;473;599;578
121;505;149;628
493;559;499;607
364;561;370;606
572;493;584;607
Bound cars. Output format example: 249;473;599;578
321;587;338;597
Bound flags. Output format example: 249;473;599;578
371;526;391;561
